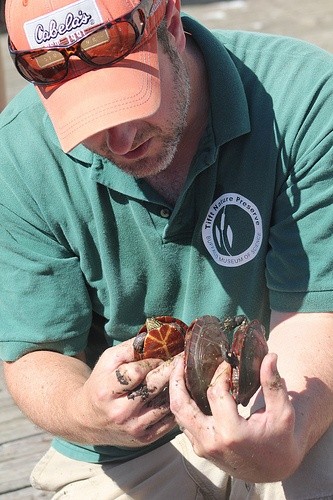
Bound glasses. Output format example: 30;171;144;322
7;0;157;84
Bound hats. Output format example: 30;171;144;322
5;0;171;152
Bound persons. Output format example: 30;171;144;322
0;0;333;500
